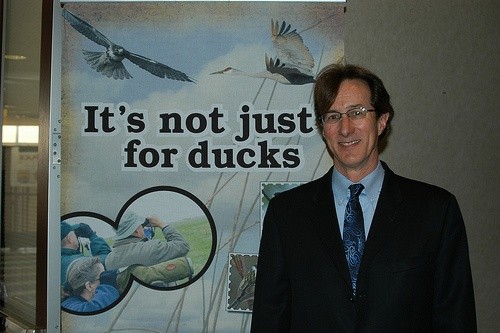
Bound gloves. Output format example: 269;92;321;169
74;222;94;238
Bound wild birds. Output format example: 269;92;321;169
209;17;317;86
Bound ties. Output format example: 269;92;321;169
342;183;366;298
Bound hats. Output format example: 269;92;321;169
61;221;80;240
113;211;146;239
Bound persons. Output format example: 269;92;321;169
250;64;478;333
59;209;192;312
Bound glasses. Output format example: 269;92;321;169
318;108;378;123
